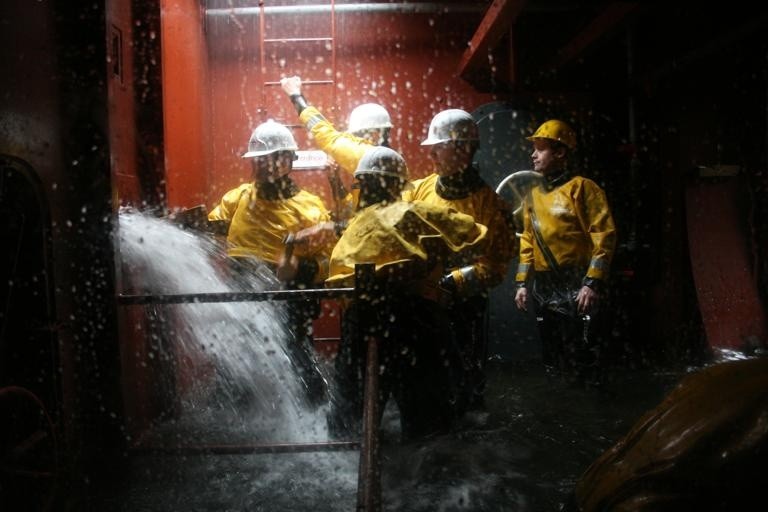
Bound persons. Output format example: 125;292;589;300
403;109;509;412
209;117;335;410
282;74;394;240
514;120;617;392
329;147;458;434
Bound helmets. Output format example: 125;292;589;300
241;122;298;158
419;109;480;147
526;121;576;150
352;146;417;192
347;104;394;133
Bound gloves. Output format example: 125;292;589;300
281;75;300;96
